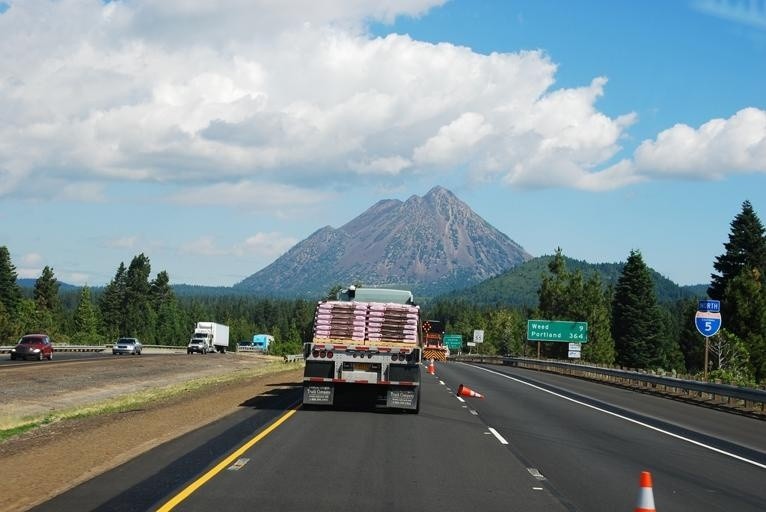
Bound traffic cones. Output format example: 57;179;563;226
632;470;659;512
457;382;485;398
426;357;436;373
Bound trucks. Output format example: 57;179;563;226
251;334;275;352
300;285;423;415
194;321;229;354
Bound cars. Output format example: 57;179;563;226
112;337;143;355
10;334;54;361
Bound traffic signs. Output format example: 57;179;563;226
694;310;723;338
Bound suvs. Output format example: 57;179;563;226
187;337;208;355
